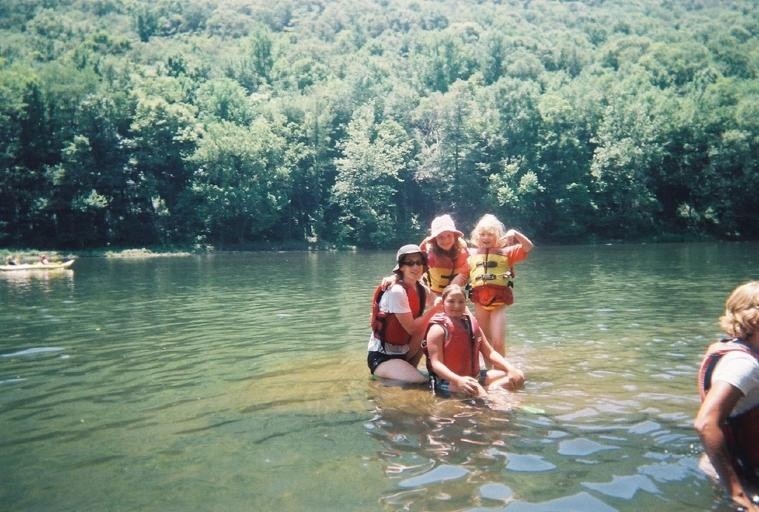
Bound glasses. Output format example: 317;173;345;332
400;260;424;265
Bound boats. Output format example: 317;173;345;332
0;259;78;271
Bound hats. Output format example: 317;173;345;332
425;213;465;243
392;243;428;275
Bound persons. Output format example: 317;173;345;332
383;213;469;312
420;284;525;397
694;282;759;512
40;255;48;265
367;245;444;383
466;213;533;359
7;256;15;265
